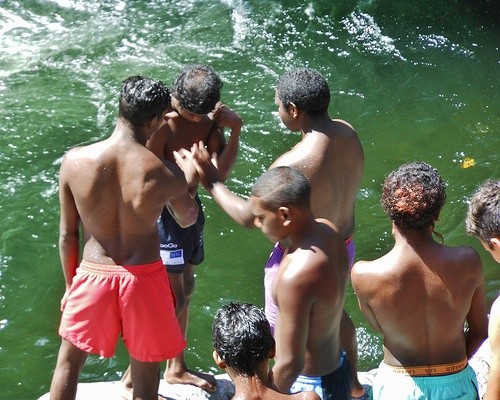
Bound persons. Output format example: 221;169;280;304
349;160;489;400
249;164;355;400
466;178;500;400
48;74;199;400
212;301;322;400
190;65;367;400
120;64;244;400
